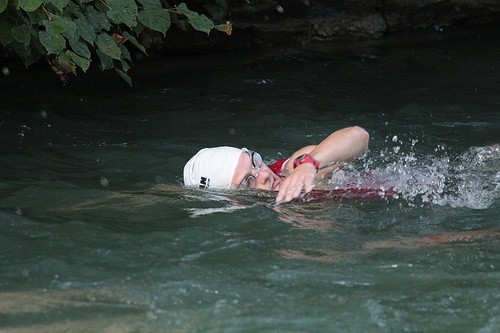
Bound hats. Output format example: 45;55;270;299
183;145;247;190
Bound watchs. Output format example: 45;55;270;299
293;153;319;175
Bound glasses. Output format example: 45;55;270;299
237;150;262;190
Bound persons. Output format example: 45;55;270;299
183;125;369;206
